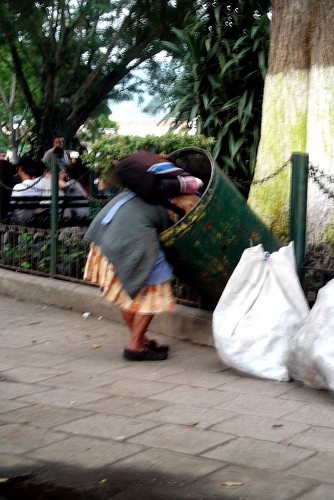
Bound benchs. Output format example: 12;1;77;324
1;193;115;226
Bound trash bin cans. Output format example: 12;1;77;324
158;146;282;309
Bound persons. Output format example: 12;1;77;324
82;193;202;361
10;136;89;224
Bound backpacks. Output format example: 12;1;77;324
112;153;204;212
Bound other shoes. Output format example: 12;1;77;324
123;348;168;361
145;340;170;351
